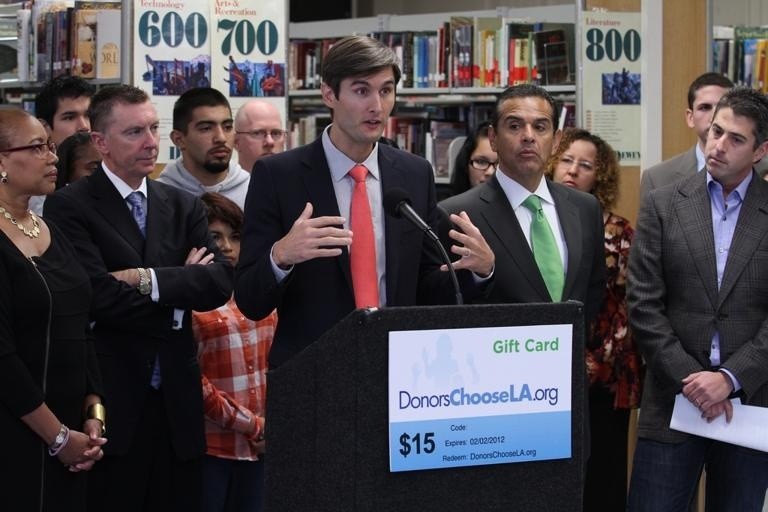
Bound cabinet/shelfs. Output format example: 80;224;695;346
0;0;130;116
285;0;578;183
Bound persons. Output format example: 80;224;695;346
447;123;500;196
433;82;608;478
543;126;647;512
1;72;286;512
233;37;505;368
642;72;768;206
627;88;767;511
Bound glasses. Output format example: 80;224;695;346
557;156;592;174
1;143;57;158
467;157;498;171
236;128;289;140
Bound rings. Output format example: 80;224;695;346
694;398;699;406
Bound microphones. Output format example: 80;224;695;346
382;189;464;303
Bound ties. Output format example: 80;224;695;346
126;192;161;391
348;165;379;309
523;195;565;303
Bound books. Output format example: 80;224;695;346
290;100;575;181
0;0;122;81
288;16;577;89
712;21;768;94
